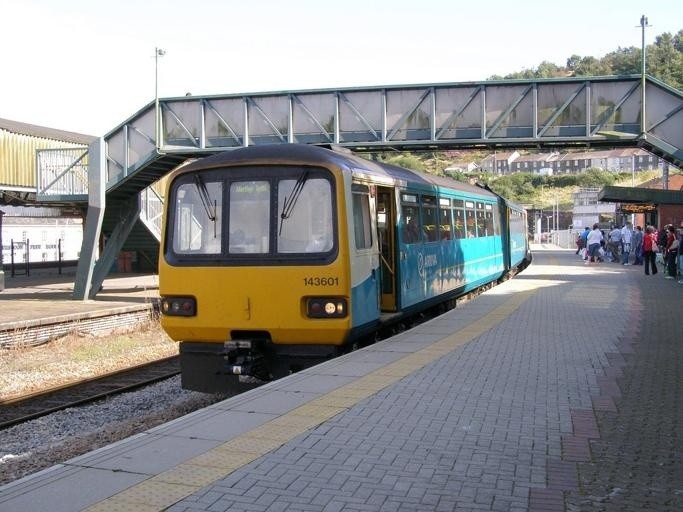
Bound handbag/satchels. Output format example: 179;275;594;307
669;240;679;250
652;240;660;252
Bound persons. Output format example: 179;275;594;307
410;223;427;243
576;221;683;285
441;219;463;241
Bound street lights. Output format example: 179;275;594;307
154;45;166;153
638;16;648;140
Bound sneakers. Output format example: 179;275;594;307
664;276;674;279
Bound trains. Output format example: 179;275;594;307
158;142;532;395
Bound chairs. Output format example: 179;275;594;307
424;224;484;243
